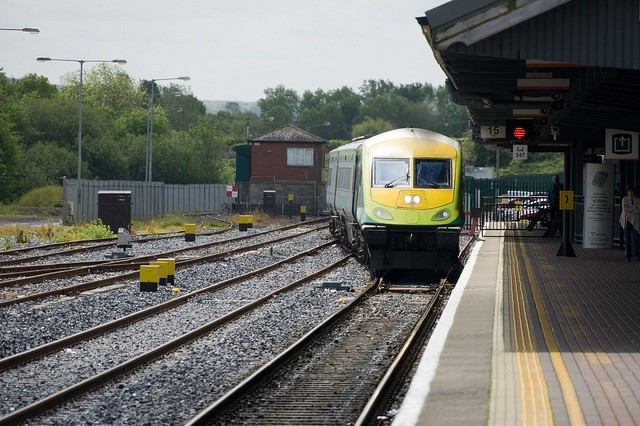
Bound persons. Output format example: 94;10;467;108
548;175;564;234
618;187;640;263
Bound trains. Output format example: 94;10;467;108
324;127;462;272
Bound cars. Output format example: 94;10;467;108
501;195;550;221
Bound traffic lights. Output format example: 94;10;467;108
507;120;530;141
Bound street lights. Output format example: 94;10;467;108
0;28;40;34
36;57;128;180
148;76;190;181
